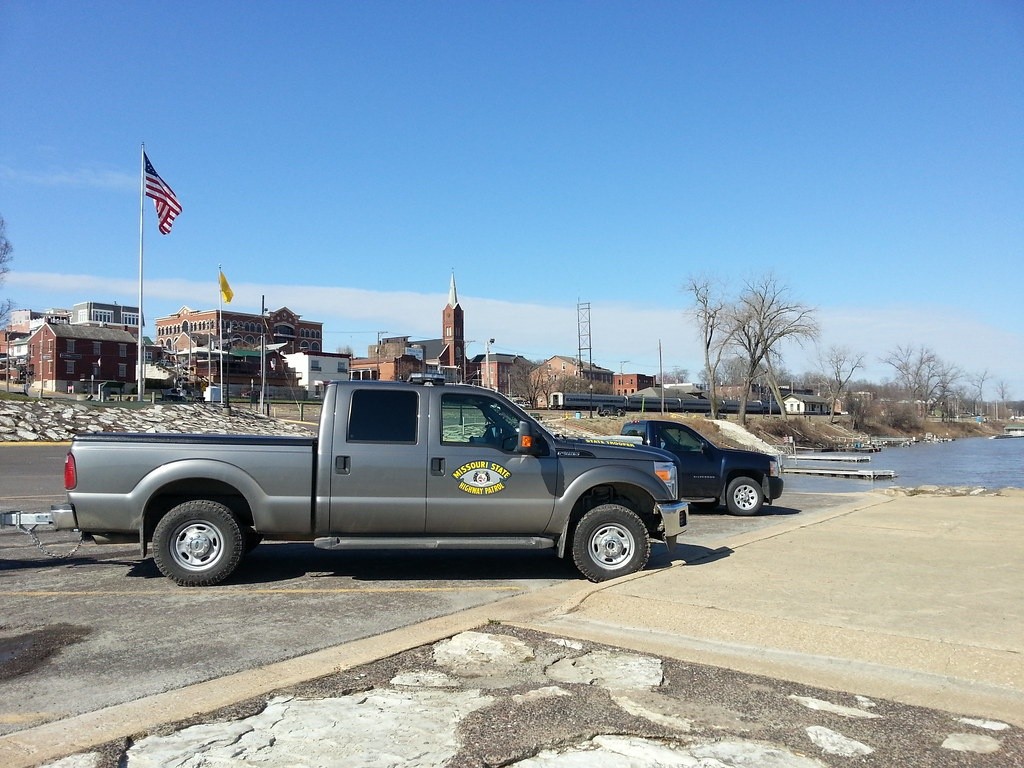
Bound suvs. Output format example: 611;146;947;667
596;403;626;417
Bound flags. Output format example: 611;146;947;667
219;271;234;304
144;152;182;237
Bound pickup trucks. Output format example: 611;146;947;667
61;373;689;585
574;420;783;515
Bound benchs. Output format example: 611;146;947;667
110;394;140;402
160;388;183;402
190;391;206;404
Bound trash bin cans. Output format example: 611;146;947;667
575;411;581;419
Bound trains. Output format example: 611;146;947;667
549;392;784;415
